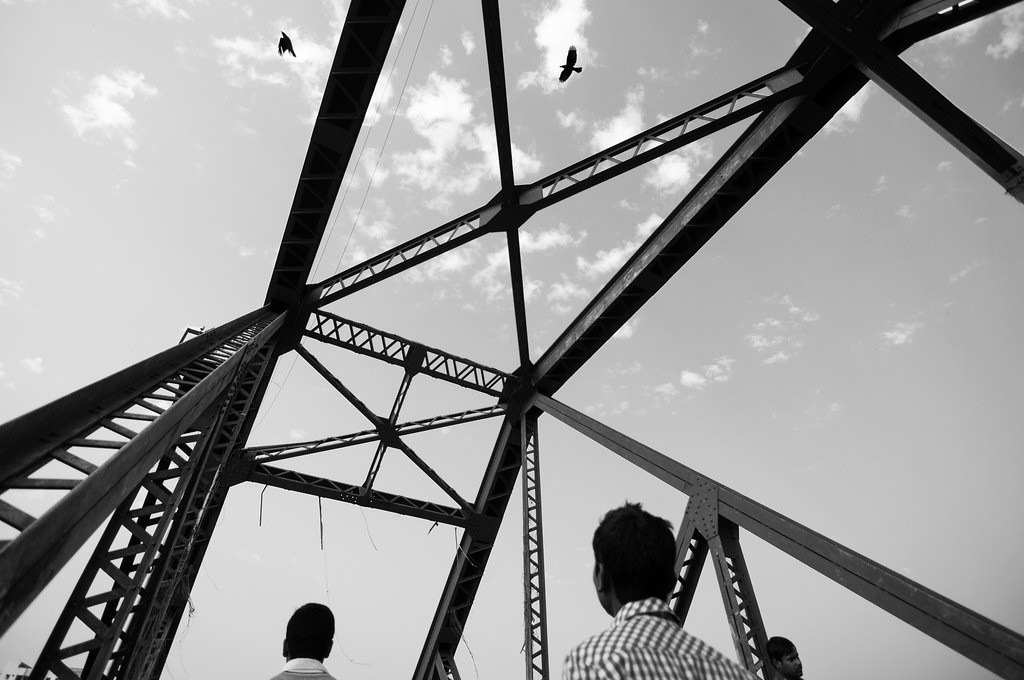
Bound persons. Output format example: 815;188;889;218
270;602;335;680
766;637;804;680
563;501;761;680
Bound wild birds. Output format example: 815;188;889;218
278;31;296;57
559;46;582;83
